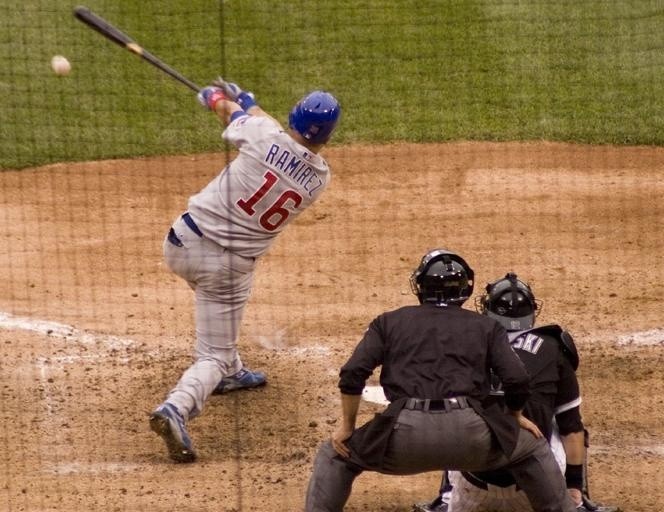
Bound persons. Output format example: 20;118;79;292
412;269;622;510
145;73;341;463
304;248;580;511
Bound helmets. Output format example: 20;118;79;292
480;272;539;331
286;89;341;145
408;250;476;308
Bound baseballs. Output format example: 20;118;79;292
52;55;68;70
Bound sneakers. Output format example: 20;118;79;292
148;402;199;464
213;366;270;395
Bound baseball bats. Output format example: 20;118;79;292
74;7;201;93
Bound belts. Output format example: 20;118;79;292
181;212;203;238
402;397;474;412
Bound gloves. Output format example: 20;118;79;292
212;76;257;106
198;85;227;110
